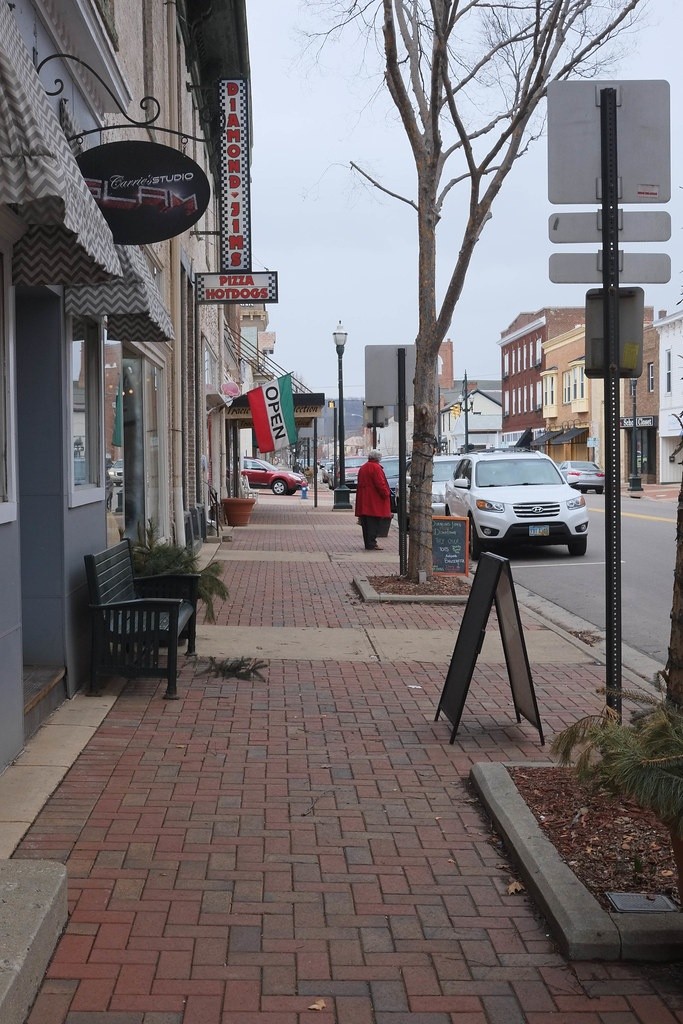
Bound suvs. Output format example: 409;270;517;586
237;457;307;495
445;449;589;561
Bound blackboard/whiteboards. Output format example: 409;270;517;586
439;552;544;731
432;515;469;577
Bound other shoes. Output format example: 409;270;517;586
373;544;383;550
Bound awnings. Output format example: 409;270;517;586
529;430;563;446
551;428;587;444
516;427;532;447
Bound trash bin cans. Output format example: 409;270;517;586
292;465;299;473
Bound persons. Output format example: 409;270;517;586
355;449;392;550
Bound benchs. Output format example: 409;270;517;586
82;536;201;701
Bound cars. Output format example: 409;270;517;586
317;459;333;489
108;459;124;486
395;453;461;516
295;459;315;469
559;461;605;494
331;456;368;489
379;456;398;514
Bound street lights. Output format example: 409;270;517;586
458;369;474;453
332;321;353;510
628;378;644;492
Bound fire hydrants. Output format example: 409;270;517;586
301;481;307;499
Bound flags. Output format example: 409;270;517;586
247;374;298;454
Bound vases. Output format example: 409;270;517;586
221;498;257;525
